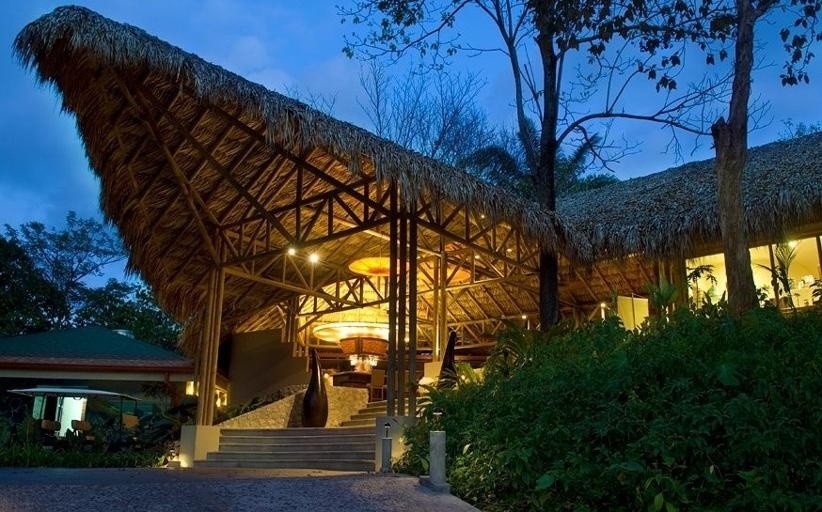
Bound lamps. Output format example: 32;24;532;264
312;307;413;376
348;235;412;277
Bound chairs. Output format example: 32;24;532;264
366;369;385;402
42;420;92;436
395;371;410;397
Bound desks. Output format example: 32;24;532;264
791;287;814;307
